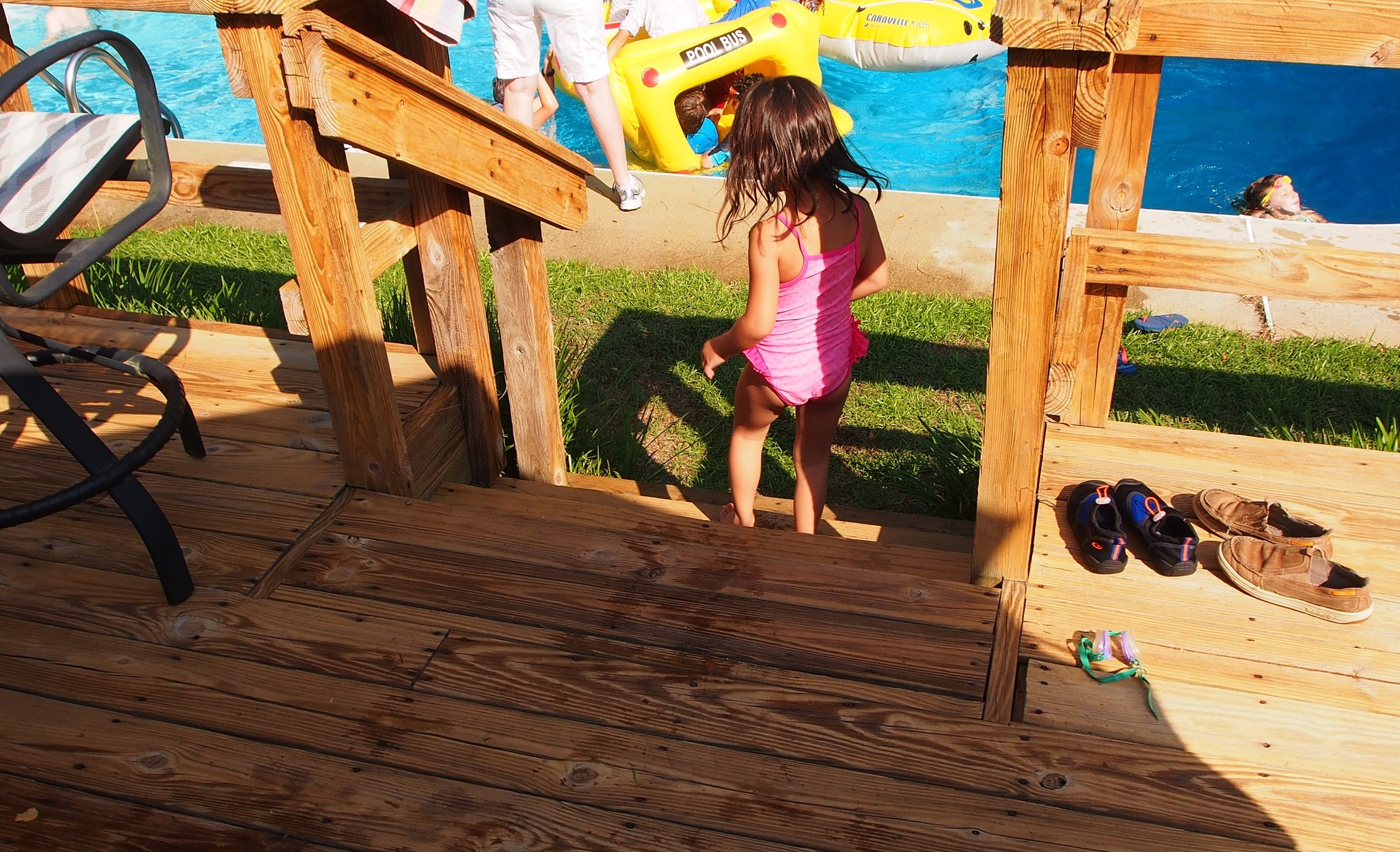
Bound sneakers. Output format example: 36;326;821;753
1067;480;1128;574
613;172;644;211
1115;477;1199;576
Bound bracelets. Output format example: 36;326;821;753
541;68;555;77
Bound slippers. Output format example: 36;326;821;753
1115;344;1137;373
1131;313;1187;334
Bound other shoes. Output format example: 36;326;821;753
1192;488;1333;560
1216;535;1373;624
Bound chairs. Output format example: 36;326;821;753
1;31;206;608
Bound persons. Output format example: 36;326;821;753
487;0;645;210
701;76;890;535
710;0;771;24
607;0;709;61
1248;174;1300;211
491;44;559;129
45;5;89;33
701;73;765;168
674;86;718;153
799;0;825;15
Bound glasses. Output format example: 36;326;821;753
1274;176;1293;186
1091;629;1139;663
